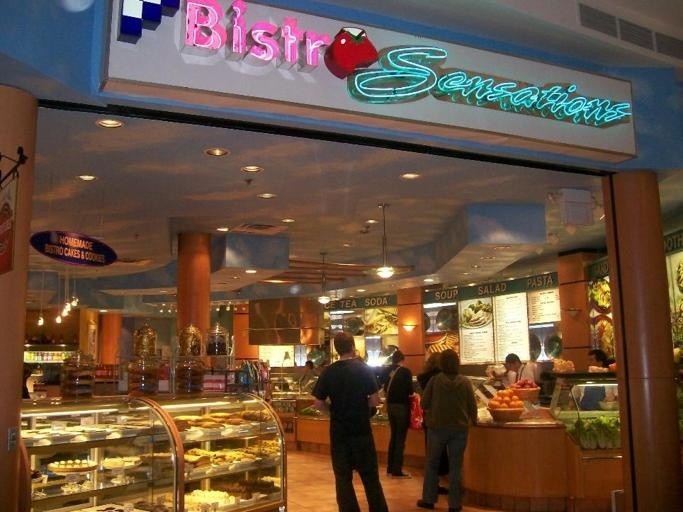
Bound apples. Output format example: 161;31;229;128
332;27;378;74
509;378;537;388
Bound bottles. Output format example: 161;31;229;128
132;318;158;354
125;351;161;398
61;349;97;402
206;319;227;356
173;351;204;400
178;321;203;356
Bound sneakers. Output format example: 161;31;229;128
436;486;449;494
414;498;465;512
385;470;412;479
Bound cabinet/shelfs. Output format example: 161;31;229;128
16;390;296;512
540;368;620;453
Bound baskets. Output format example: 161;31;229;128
488;408;525;422
510;387;541;405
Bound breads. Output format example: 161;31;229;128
238;407;283;497
20;413;256;512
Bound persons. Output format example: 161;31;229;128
416;351;450;496
311;331;390;512
505;354;536;385
378;349;415;479
586;348;609;370
416;348;478;512
487;363;518;390
297;361;318;385
